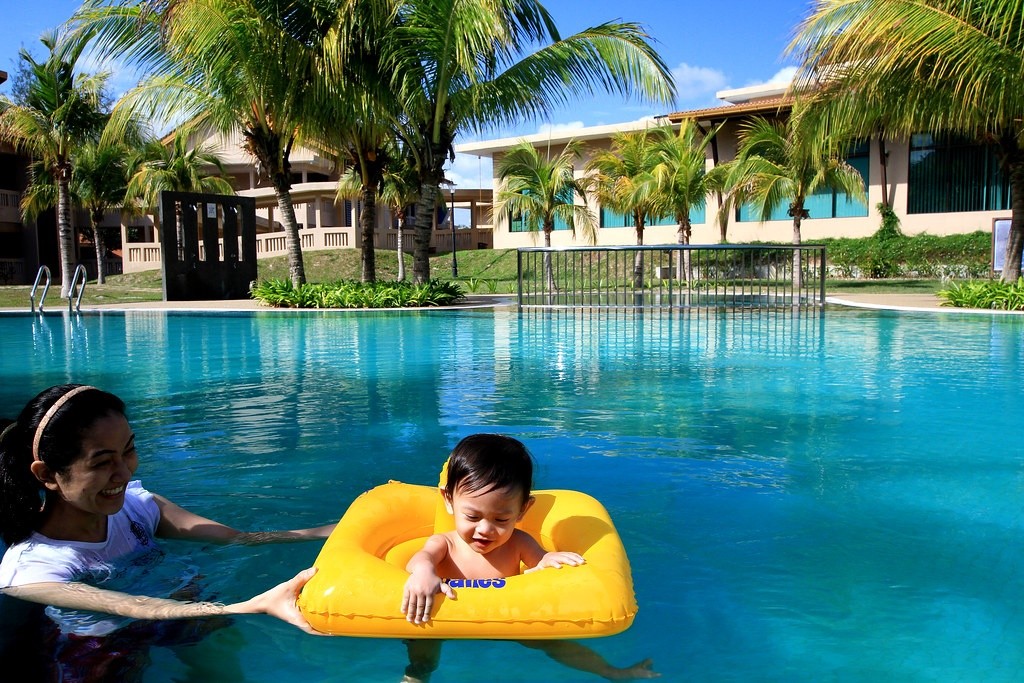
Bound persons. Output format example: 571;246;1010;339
398;433;587;624
0;381;345;636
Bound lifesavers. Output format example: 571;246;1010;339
296;450;639;641
